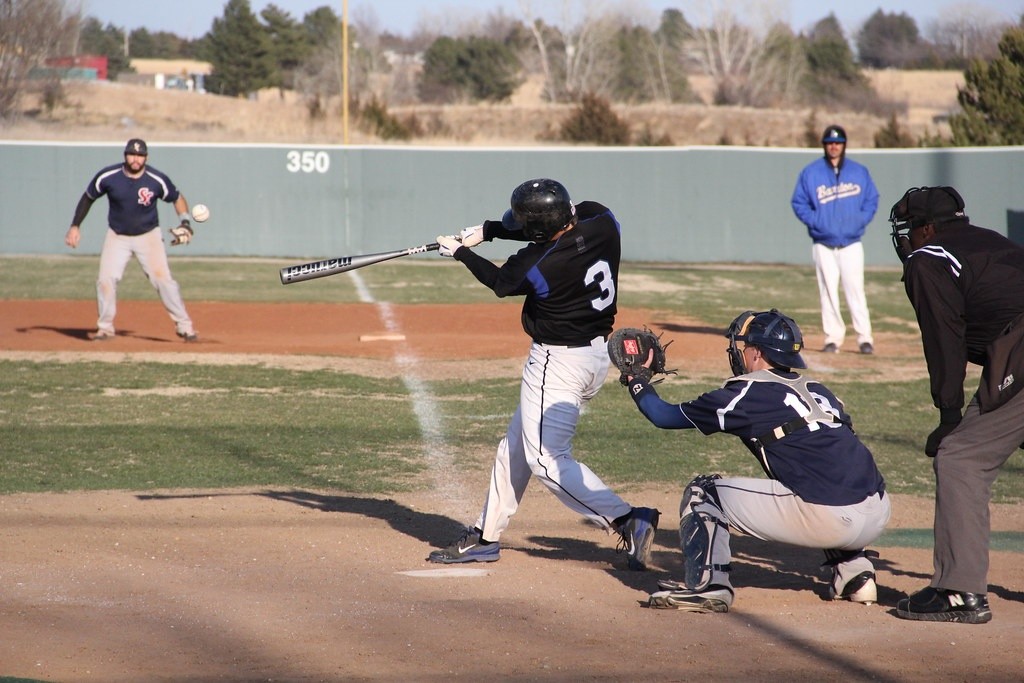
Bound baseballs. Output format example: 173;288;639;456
192;204;210;223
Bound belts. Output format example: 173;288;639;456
569;335;609;349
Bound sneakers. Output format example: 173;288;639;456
613;506;660;572
429;523;500;562
896;582;999;619
649;585;732;612
822;575;878;606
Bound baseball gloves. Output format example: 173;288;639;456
169;224;194;247
607;330;667;388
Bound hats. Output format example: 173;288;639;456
821;126;846;143
125;139;147;156
896;186;967;226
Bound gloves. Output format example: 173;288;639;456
924;422;956;457
460;225;487;248
436;235;464;260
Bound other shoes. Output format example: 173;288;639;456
824;343;838;357
176;330;197;341
861;341;873;356
92;329;110;341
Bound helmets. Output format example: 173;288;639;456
748;312;808;369
502;178;573;236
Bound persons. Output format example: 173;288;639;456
888;186;1024;624
618;308;891;614
790;125;880;353
65;138;194;342
429;180;658;570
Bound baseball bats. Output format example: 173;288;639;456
279;235;464;286
